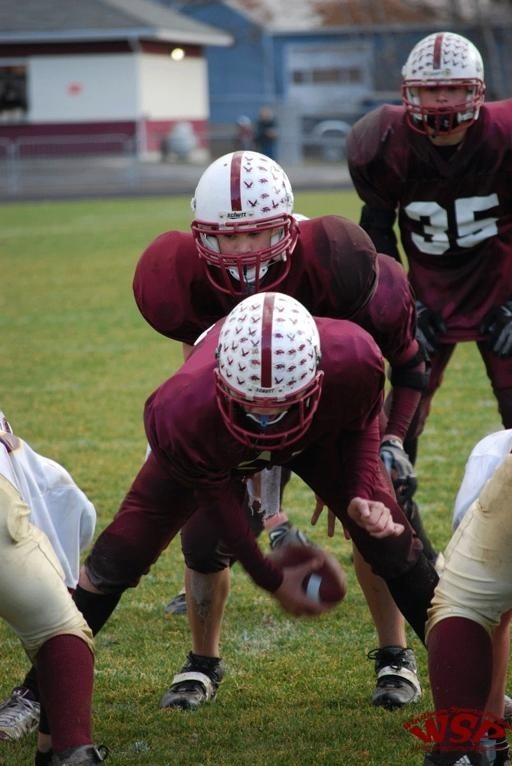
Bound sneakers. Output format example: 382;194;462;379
0;588;511;766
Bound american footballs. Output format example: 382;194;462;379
264;545;347;606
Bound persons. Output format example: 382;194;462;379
451;430;511;763
135;146;429;708
161;105;281;168
2;411;107;766
427;428;511;763
2;284;509;747
345;30;512;479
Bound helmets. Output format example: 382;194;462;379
190;150;301;298
214;292;325;451
401;32;486;136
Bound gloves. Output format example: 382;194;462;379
408;299;447;366
478;300;512;358
269;520;307;550
379;439;417;519
